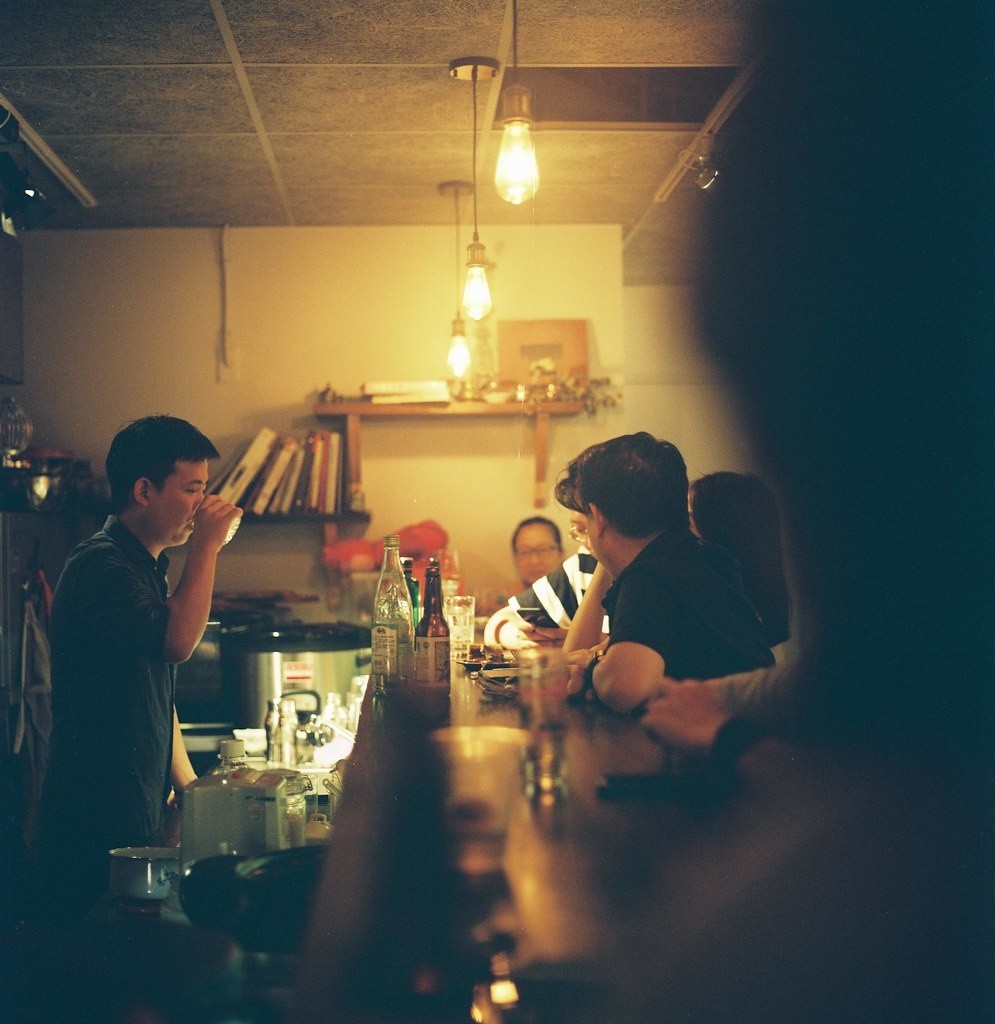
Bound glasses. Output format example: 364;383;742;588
518;545;558;558
569;526;588;545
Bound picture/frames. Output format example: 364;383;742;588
498;318;588;389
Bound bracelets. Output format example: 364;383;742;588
584;650;606;687
495;619;509;645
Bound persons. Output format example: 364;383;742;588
698;638;800;722
554;430;776;718
641;674;760;765
481;476;610;650
475;516;564;619
687;471;791;647
27;413;244;1023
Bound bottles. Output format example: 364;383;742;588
205;740;249;776
323;692;347;727
265;697;281;762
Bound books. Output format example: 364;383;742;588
358;381;454;406
204;426;344;518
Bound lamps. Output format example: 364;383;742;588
493;0;540;205
448;55;503;323
438;179;476;382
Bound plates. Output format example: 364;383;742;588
455;660;517;672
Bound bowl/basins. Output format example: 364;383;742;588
109;847;172;898
180;845;328;951
233;729;267;756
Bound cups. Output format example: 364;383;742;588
416;566;450;697
190;503;240;546
400;557;420;627
304;772;319;820
260;769;306;848
444;596;475;660
373;536;415;695
